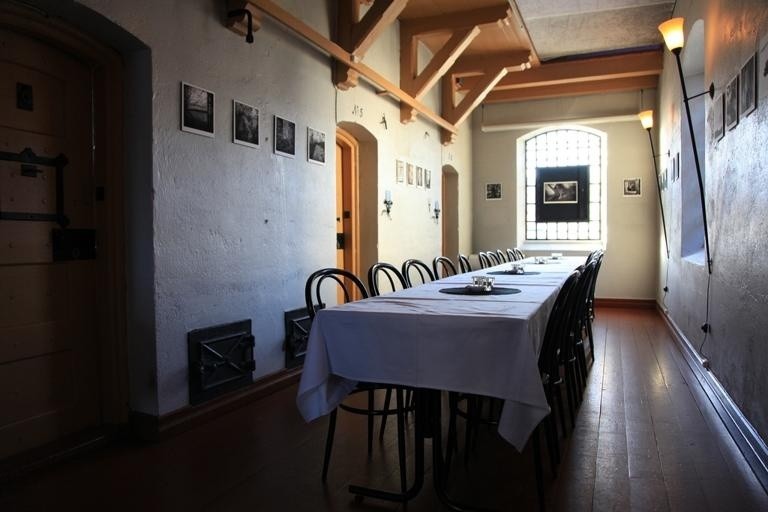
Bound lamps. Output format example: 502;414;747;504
654;14;718;276
634;107;675;261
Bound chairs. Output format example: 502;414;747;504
305;247;605;508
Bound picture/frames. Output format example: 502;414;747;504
393;158;434;192
484;181;504;202
655;151;681;194
710;55;761;144
542;180;579;206
178;78;216;141
231;96;261;152
304;124;327;167
271;113;297;161
621;177;644;198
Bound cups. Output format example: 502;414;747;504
471;275;495;292
511;253;562;274
